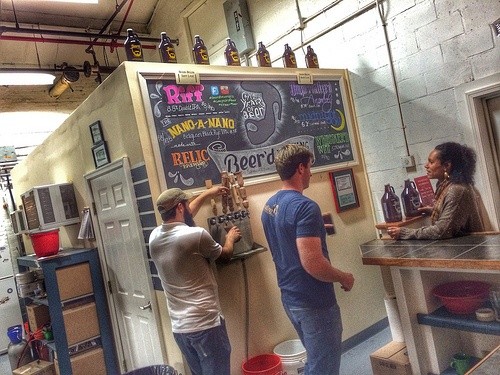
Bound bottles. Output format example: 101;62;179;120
158;32;178;63
256;42;271;67
282;43;297;68
223;38;241;67
381;184;402;224
192;34;210;65
124;28;144;62
305;45;319;68
401;179;423;219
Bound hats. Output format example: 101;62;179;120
157;187;193;215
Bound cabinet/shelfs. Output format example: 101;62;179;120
16;247;119;375
389;265;500;375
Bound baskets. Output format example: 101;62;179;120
434;280;490;315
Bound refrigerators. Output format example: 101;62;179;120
0;218;26;356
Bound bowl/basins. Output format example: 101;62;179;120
475;308;495;321
431;280;492;315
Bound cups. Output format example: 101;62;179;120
450;352;471;375
322;212;335;237
489;287;500;322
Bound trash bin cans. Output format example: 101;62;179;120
121;365;184;375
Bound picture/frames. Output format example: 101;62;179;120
88;120;111;169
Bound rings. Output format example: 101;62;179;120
389;230;392;233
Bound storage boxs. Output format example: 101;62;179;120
370;340;412;375
10;181;81;235
12;263;107;375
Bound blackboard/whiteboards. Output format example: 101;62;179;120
137;72;359;199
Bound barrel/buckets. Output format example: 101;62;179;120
241;354;283;375
273;339;307;375
29;228;60;257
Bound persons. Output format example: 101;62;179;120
149;185;243;375
387;142;484;241
261;144;354;375
